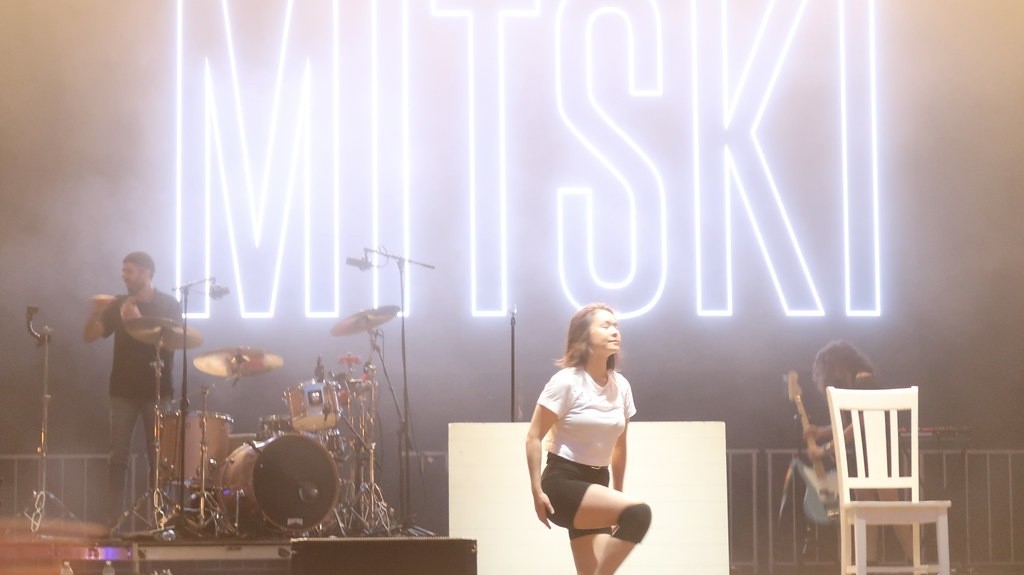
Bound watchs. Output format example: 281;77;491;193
825;442;831;450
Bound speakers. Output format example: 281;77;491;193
289;537;477;575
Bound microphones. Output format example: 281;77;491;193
315;357;324;382
209;285;229;298
368;330;379;360
347;258;373;270
308;489;320;498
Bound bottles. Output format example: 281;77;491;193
102;561;115;575
60;561;74;575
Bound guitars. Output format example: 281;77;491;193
784;370;843;524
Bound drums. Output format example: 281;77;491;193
255;415;295;444
156;409;236;490
214;429;338;534
285;377;341;432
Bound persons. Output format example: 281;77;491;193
85;251;182;536
525;305;652;575
803;341;914;575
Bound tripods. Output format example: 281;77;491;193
321;248;439;536
0;306;90;545
101;276;246;544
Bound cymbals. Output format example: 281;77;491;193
121;317;203;350
329;304;402;336
193;347;286;377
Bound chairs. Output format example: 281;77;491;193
825;385;953;575
